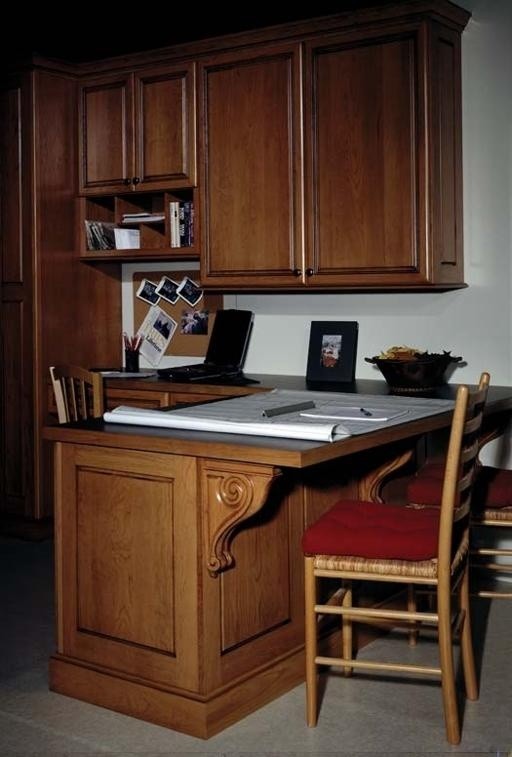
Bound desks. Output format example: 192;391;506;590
40;371;511;741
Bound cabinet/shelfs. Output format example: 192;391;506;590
202;13;466;295
76;59;201;257
1;55;122;543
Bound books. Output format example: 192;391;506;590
84;201;194;250
301;406;409;421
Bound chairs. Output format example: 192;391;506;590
301;373;490;744
408;465;511;646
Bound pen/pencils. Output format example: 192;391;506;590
360;408;371;416
123;334;144;353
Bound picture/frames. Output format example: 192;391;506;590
305;320;359;385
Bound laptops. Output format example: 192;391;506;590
156;309;255;383
